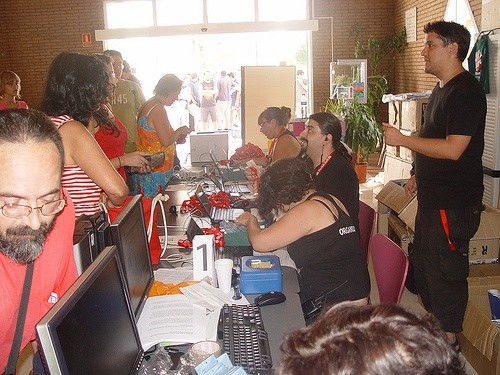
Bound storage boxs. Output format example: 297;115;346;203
374;98;500;375
190;131;229;167
239;255;283;295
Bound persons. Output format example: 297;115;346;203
275;300;467;375
86;48;241;190
234;156;372;324
0;70;29;111
299;111;360;238
0;109;79;375
381;21;487;355
40;51;129;219
240;106;303;167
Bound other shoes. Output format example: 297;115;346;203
451;337;461;354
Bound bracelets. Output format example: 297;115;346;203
116;155;122;168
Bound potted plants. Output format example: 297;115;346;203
320;25;409;182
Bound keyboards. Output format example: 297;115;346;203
217;303;275;375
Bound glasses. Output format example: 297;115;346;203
113;64;125;71
259;120;269;128
108;72;116;78
0;196;65;219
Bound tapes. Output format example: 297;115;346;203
189;340;223;365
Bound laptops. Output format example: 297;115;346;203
194;184;245;223
183;218;253;278
209;148;244;174
204;166;250;194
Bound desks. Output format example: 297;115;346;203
139;167;306;375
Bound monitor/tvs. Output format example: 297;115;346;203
108;192;154;327
34;245;145;375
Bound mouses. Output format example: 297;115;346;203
254;290;286;307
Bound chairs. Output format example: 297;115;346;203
369;233;409;306
358;199;377;264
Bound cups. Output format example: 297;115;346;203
215;258;233;291
189;341;222;375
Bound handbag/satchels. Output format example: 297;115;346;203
125;150;164;174
